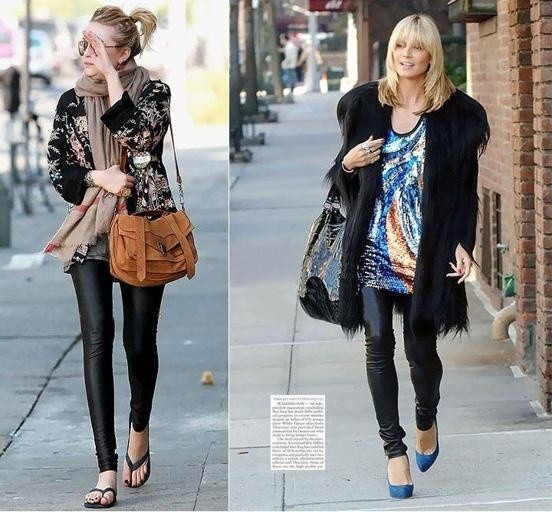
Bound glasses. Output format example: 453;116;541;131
78;39;119;56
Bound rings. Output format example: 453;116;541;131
364;143;373;159
121;187;131;198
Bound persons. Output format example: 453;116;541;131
277;30;308;103
298;15;490;497
48;5;196;508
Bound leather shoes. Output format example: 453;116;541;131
386;450;414;500
416;415;439;473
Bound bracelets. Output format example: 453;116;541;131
84;170;96;187
342;161;355;174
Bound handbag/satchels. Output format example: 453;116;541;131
295;182;363;329
107;209;198;288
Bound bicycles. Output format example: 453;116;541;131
9;107;56;217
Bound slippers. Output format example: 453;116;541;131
84;488;117;509
123;418;150;489
